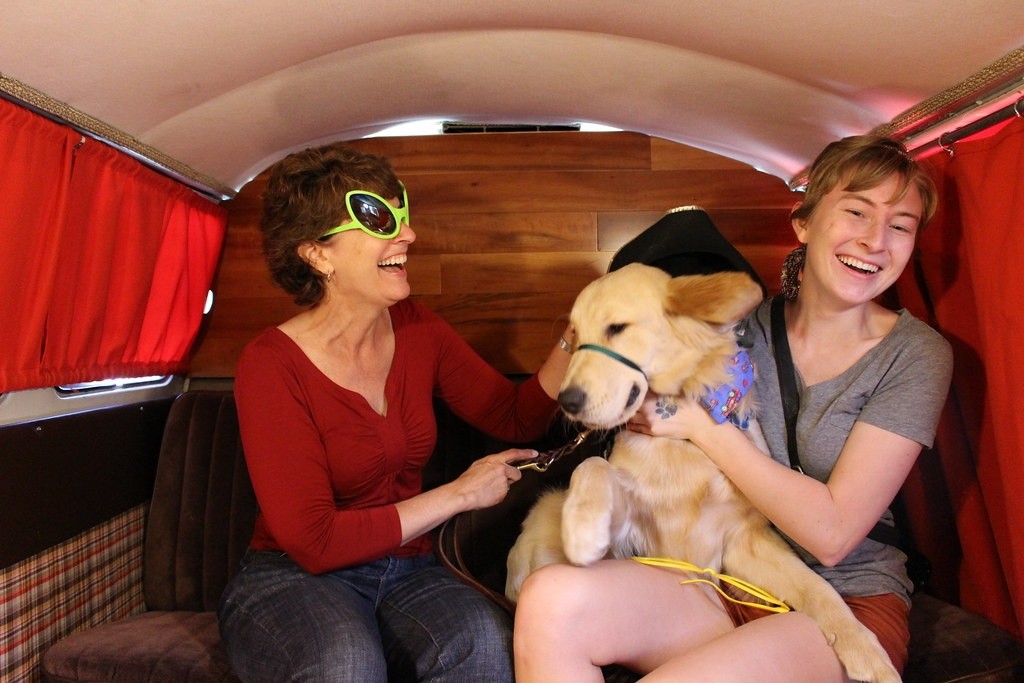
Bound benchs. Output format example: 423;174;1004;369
46;374;1024;683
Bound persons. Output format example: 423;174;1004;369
217;143;576;683
512;135;954;683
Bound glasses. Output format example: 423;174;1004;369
311;179;410;240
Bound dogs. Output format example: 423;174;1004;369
505;261;903;683
